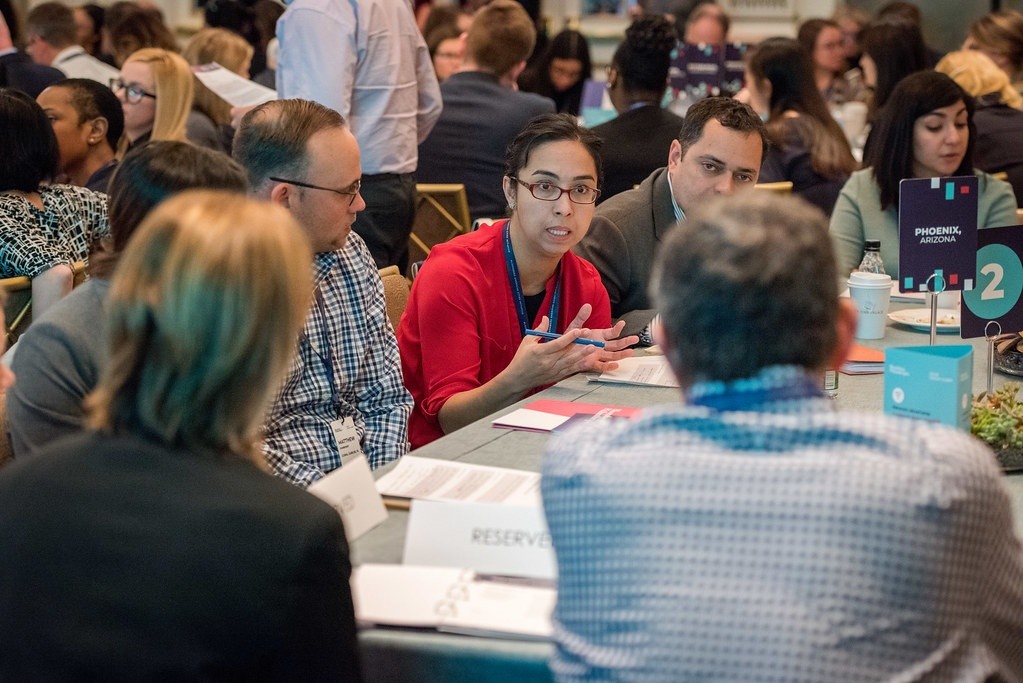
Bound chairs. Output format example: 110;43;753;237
376;264;410;332
0;261;86;351
402;183;471;281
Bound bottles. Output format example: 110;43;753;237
859;239;885;275
822;363;839;399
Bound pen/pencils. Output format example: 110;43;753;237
525;329;605;348
473;572;556;590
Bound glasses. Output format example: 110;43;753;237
509;175;602;204
109;79;157;105
269;177;360;207
604;63;614;76
23;37;47;55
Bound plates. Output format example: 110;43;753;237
888;308;962;331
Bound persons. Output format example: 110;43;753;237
0;0;1023;490
0;189;368;683
539;189;1023;682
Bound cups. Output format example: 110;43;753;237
847;272;893;339
926;291;962;311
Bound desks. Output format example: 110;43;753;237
329;277;1023;683
